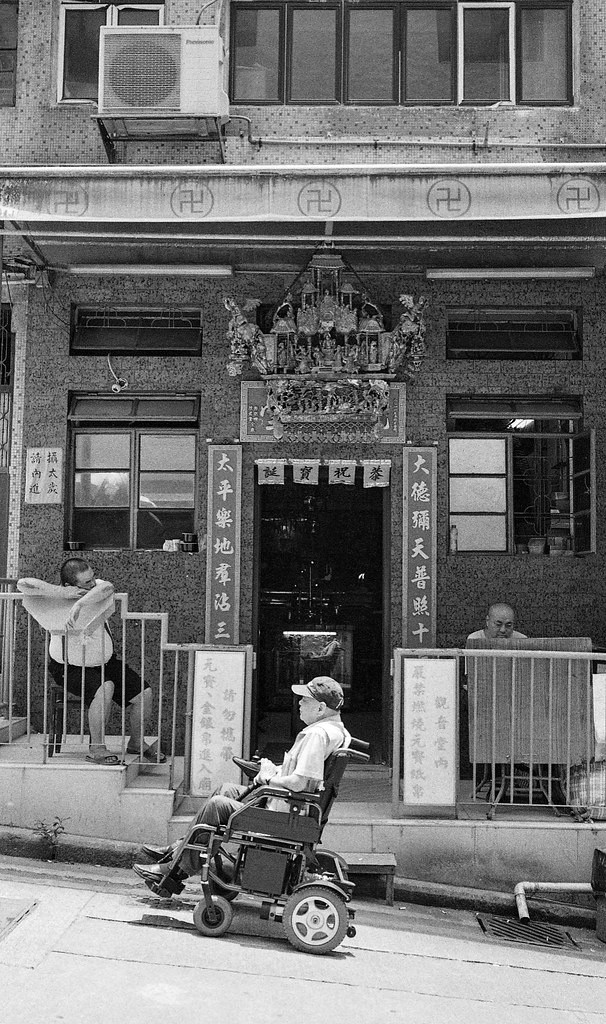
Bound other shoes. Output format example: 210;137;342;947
142;844;173;863
232;756;261;778
132;864;186;895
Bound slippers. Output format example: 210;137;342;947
126;746;168;763
85;750;119;765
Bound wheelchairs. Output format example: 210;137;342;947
145;737;372;954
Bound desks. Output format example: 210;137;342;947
464;636;595;824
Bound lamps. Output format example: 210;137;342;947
426;266;596;280
67;262;235;278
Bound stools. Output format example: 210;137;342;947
48;680;88;757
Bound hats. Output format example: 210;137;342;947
291;677;345;712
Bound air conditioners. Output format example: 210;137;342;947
96;23;232;136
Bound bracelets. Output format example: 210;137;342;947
265;778;271;785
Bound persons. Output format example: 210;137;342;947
17;558;169;766
463;603;538;789
309;635;342;659
132;676;352;895
